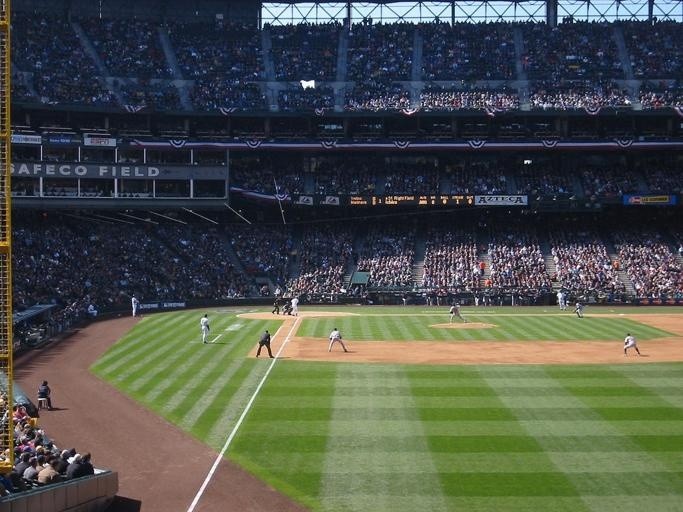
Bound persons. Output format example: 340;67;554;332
0;380;93;497
0;13;683;365
